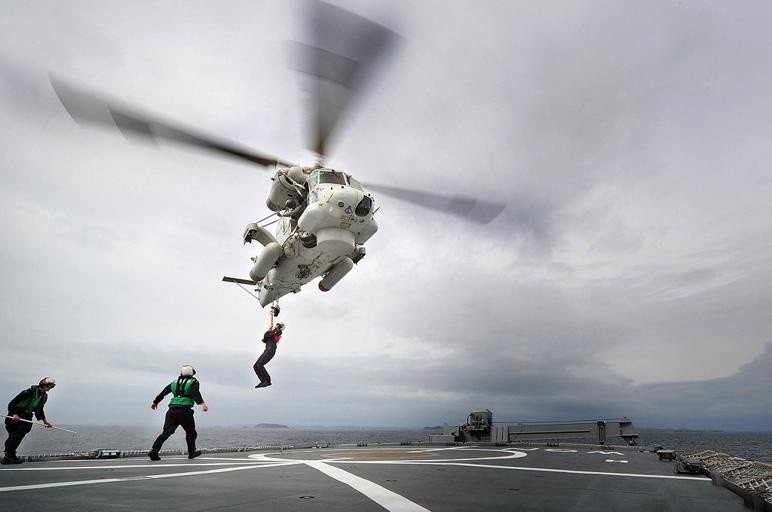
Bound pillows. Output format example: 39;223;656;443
40;377;56;386
277;321;285;331
180;365;196;376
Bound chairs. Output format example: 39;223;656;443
188;450;201;460
1;452;22;464
255;381;272;388
148;450;161;462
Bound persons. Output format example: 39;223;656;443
253;321;286;388
148;365;208;461
1;376;56;465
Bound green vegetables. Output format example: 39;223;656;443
45;1;507;316
